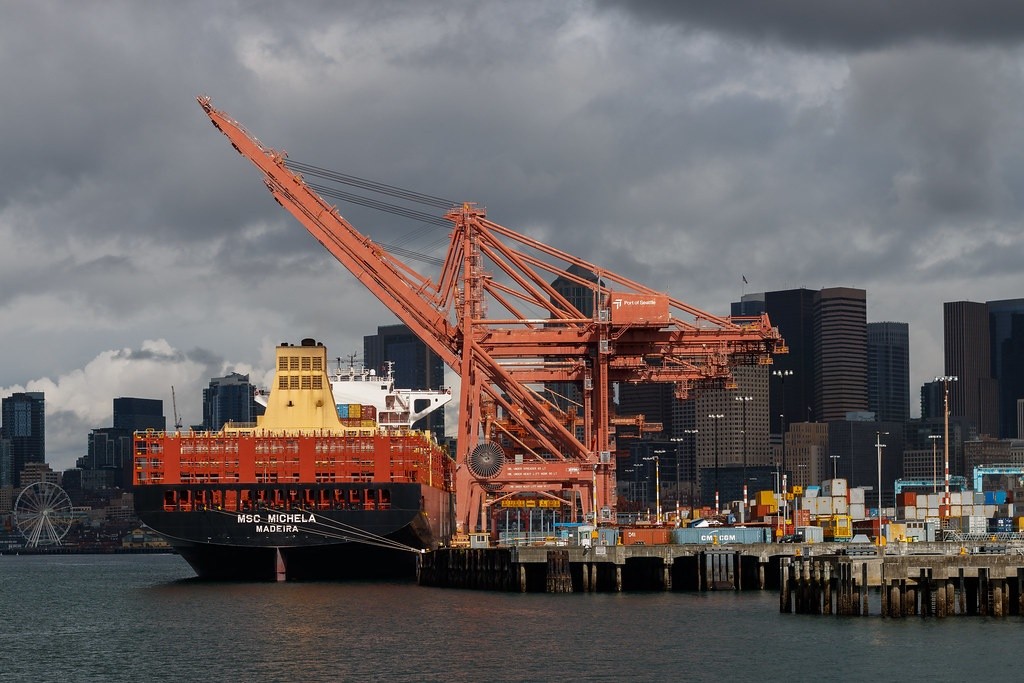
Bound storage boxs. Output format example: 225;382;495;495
336;404;409;436
596;478;1024;546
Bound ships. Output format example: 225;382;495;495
130;338;458;579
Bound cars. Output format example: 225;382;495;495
779;533;802;543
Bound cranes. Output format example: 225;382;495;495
190;92;792;547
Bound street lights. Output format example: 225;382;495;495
934;375;958;540
877;430;890;545
708;413;726;518
624;437;684;524
830;456;840;479
928;435;942;493
770;368;794;537
735;395;754;525
684;429;700;520
